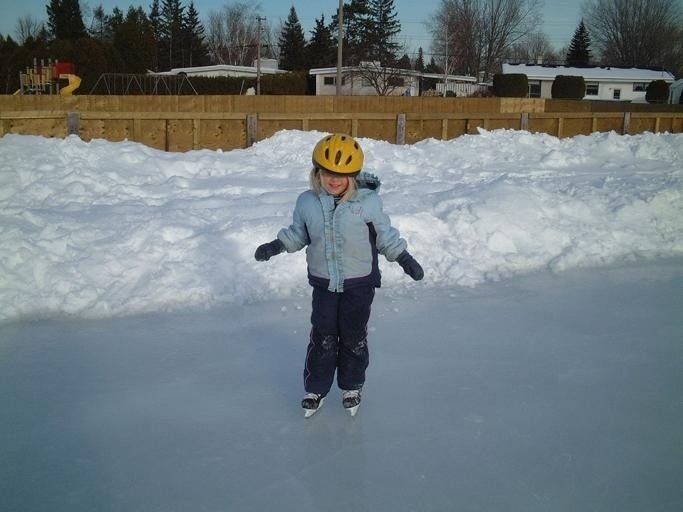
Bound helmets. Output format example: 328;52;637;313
310;132;365;177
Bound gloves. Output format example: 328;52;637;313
253;238;288;262
395;249;424;282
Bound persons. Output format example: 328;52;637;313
254;132;426;411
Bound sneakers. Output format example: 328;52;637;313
301;391;327;410
340;387;362;409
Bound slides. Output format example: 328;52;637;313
13;89;20;95
60;74;81;95
